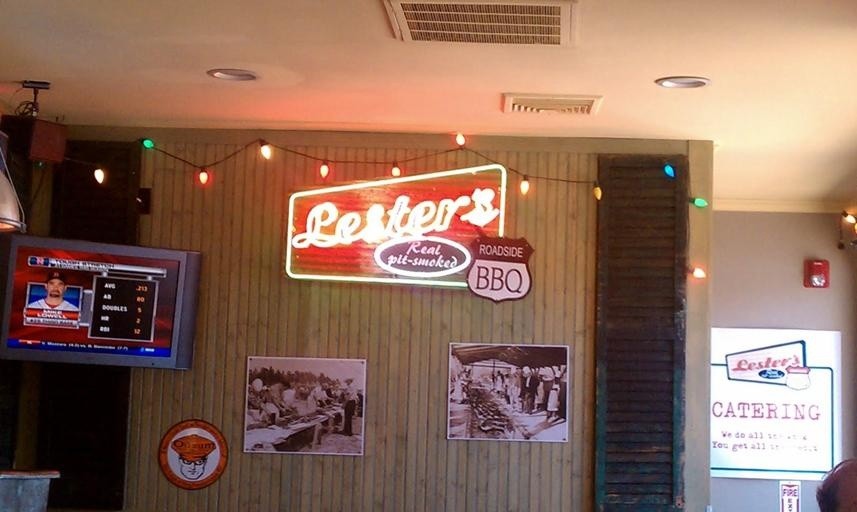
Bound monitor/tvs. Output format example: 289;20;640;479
0;234;201;370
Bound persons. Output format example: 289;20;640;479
249;369;359;435
454;365;567;422
26;273;79;320
815;457;856;512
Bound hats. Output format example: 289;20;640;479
47;271;66;284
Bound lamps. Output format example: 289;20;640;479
51;126;716;287
835;209;857;252
